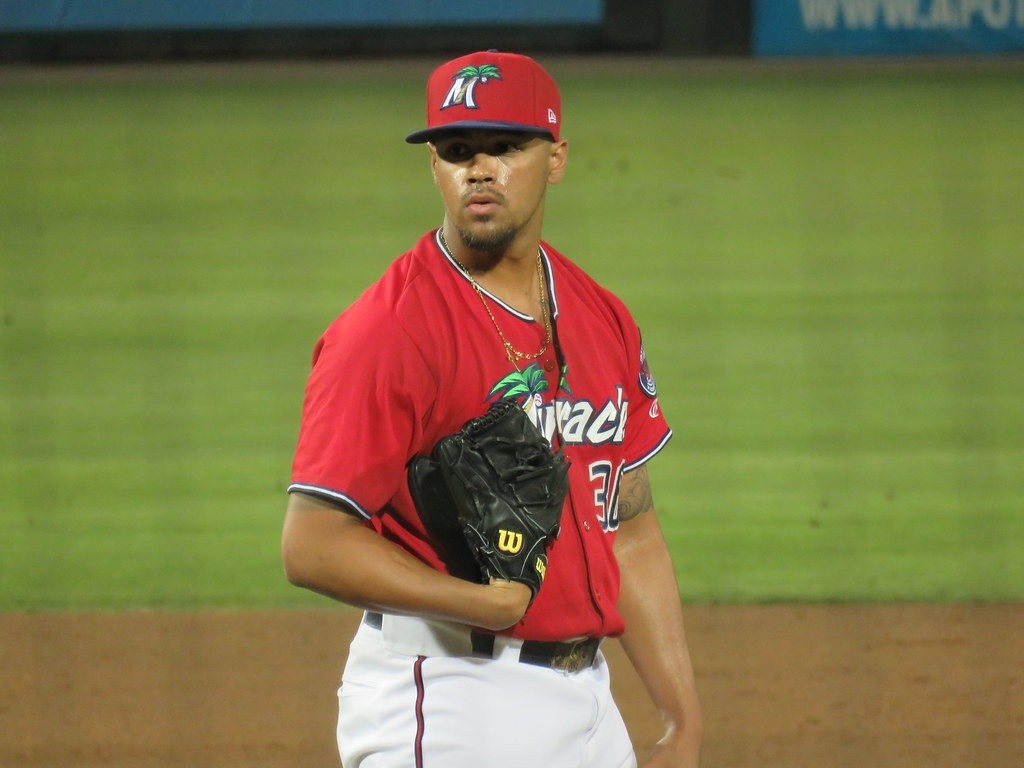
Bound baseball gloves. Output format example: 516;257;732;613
405;395;573;604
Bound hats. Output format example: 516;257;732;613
405;48;562;145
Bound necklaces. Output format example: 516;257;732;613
438;230;551;358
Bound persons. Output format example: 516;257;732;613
282;48;703;768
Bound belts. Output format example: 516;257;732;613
363;609;604;672
